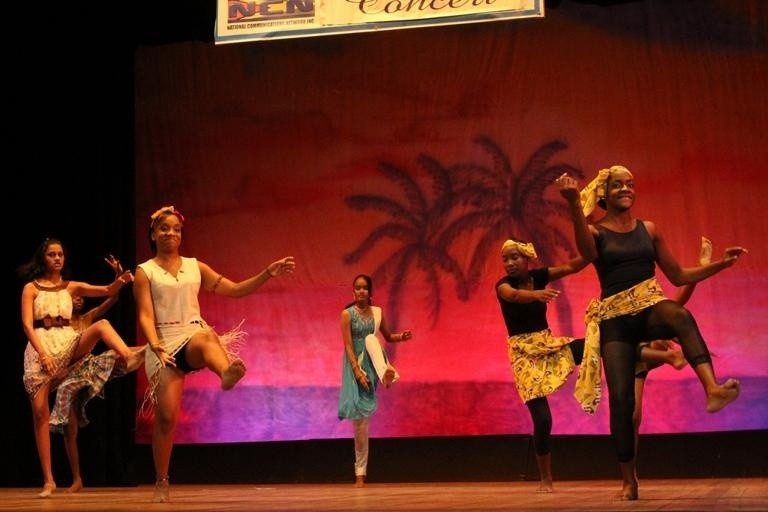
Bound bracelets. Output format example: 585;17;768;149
151;343;160;346
266;268;273;277
356;372;362;380
40;356;48;364
400;333;403;341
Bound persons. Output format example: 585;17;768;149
21;239;146;497
337;274;412;486
632;236;713;487
48;254;145;493
133;205;296;503
495;237;689;493
556;165;749;501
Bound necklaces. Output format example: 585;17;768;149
354;305;369;314
